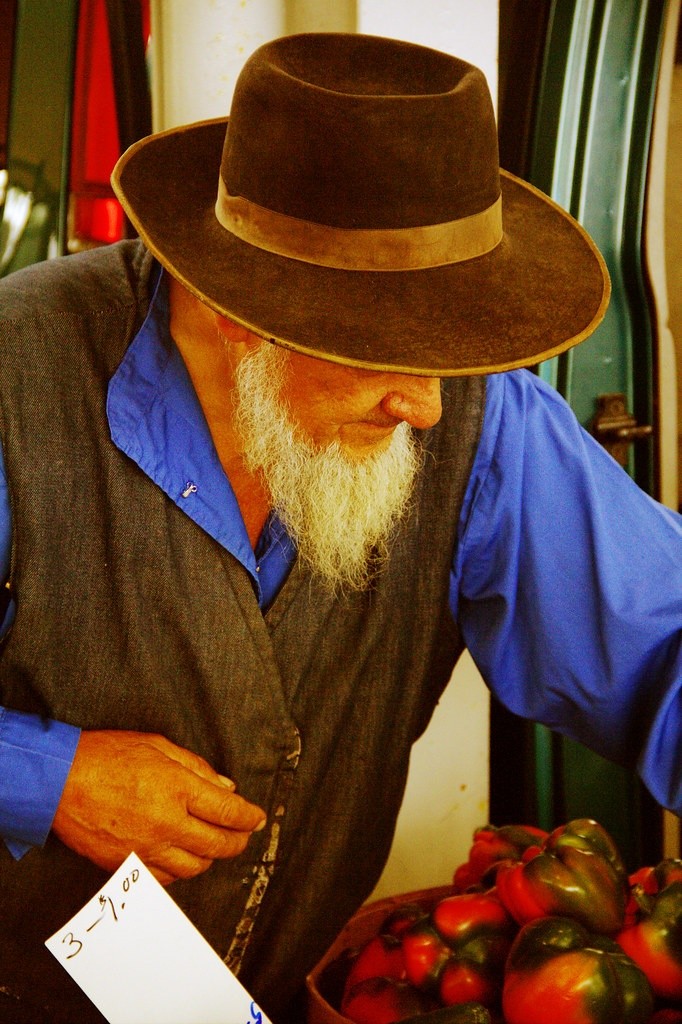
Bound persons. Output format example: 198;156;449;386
1;31;682;1023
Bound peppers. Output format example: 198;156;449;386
341;819;682;1024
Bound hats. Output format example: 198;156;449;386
108;32;611;378
